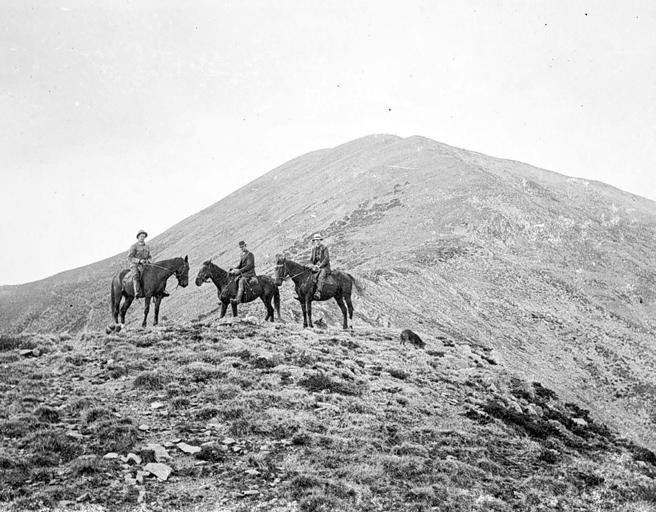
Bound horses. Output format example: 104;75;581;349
274;256;368;336
195;257;282;325
108;254;190;329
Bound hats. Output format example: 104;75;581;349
134;229;148;239
311;233;324;242
237;240;246;248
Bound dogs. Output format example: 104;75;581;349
398;328;427;350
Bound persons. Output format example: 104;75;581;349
127;229;153;299
292;233;331;300
226;240;255;304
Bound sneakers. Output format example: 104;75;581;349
313;291;321;300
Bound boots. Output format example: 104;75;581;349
133;279;170;299
229;288;244;304
